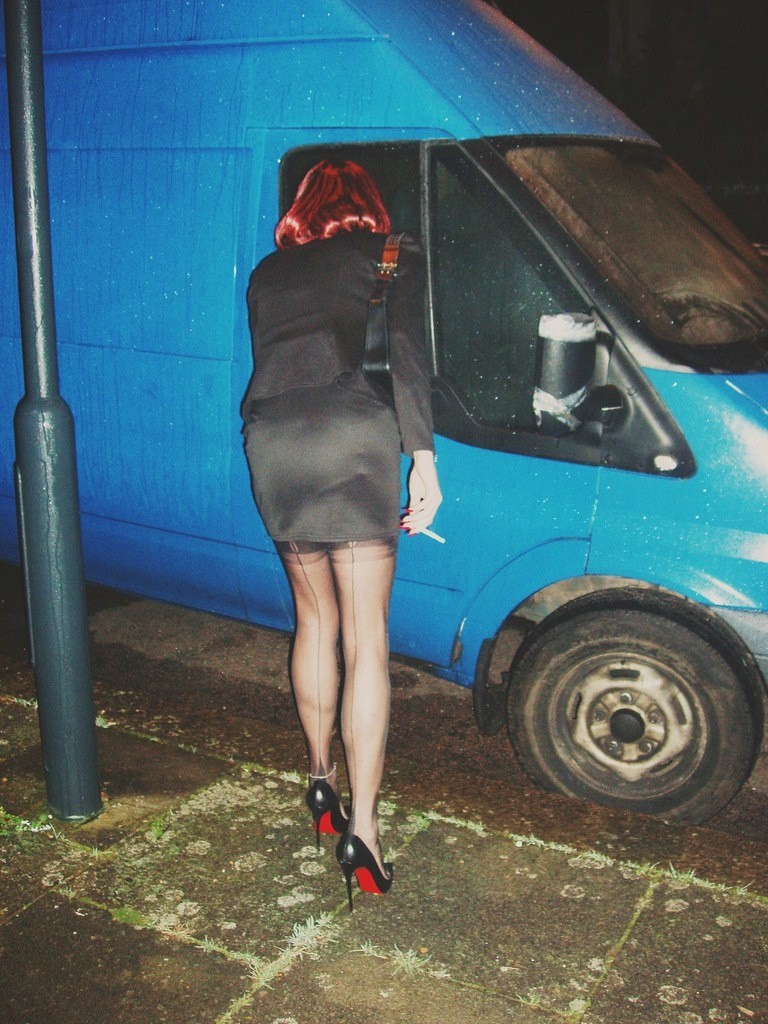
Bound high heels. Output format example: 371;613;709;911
336;834;392;911
306;779;351;848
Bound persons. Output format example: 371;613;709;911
241;158;442;914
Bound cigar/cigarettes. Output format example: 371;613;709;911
420;527;446;545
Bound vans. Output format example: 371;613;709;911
0;0;768;828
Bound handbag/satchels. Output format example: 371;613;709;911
362;232;405;410
